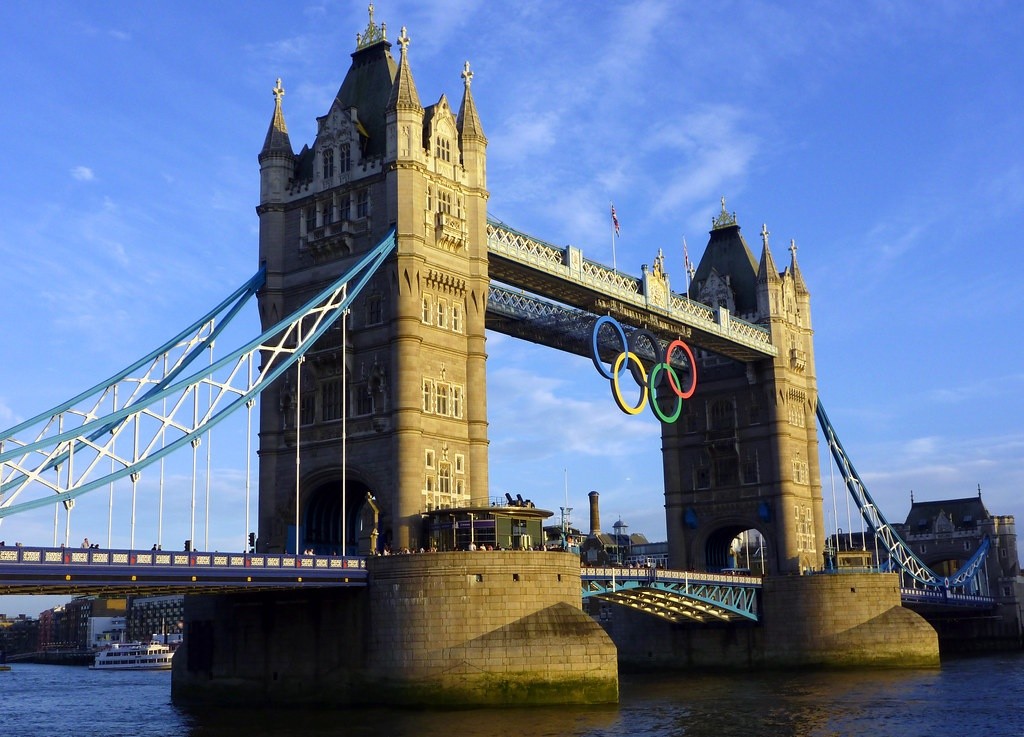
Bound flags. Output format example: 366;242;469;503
612;205;620;238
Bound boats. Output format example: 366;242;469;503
88;640;175;670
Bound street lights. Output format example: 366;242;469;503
467;512;475;544
449;513;456;548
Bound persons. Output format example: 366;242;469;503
151;544;157;551
303;548;314;555
81;538;99;549
580;559;664;570
374;541;561;556
